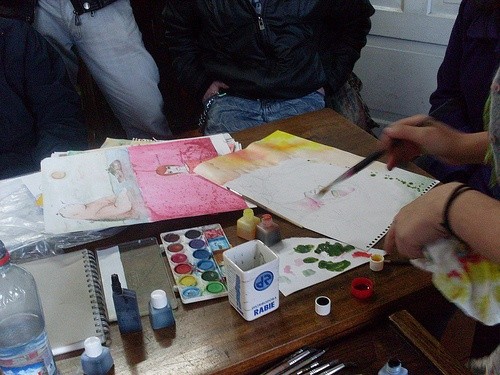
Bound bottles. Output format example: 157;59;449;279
0;240;61;375
378;358;409;375
237;209;260;242
255;214;283;245
145;290;173;331
80;336;115;375
111;273;143;335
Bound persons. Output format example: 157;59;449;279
0;0;376;180
407;0;500;359
379;67;500;267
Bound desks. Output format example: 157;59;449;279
52;107;475;375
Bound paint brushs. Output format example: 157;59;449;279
260;345;318;375
281;347;330;375
225;185;303;229
316;98;453;196
301;358;346;375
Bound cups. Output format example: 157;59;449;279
222;240;279;322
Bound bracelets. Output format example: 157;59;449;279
443;185;479;246
